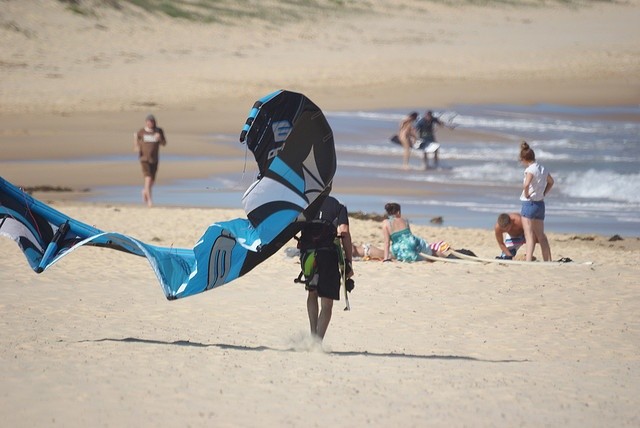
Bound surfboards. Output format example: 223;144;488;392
388;133;440;153
338;228;354;310
451;250;592;266
419;252;475;263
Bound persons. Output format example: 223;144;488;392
519;141;555;262
382;203;432;263
135;114;167;209
398;110;456;171
494;213;552;261
352;243;396;258
299;195;355;342
